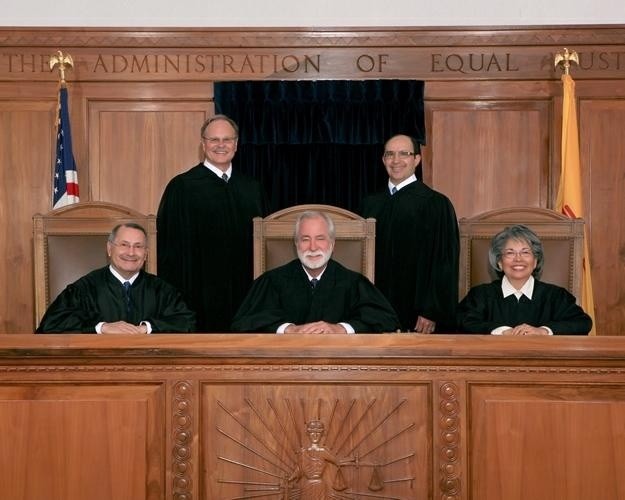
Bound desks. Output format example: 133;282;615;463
2;332;625;500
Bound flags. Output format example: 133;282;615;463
551;72;597;336
49;80;80;210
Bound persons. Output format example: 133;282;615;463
35;222;199;333
359;132;458;335
459;224;592;336
229;210;401;333
159;114;262;327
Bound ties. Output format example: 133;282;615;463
310;278;320;296
121;281;135;319
221;172;230;184
391;186;399;197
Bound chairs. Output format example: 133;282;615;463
30;197;159;331
249;204;378;289
454;203;588;307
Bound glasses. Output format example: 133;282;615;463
383;150;419;159
201;134;238;144
107;238;148;250
498;250;536;257
